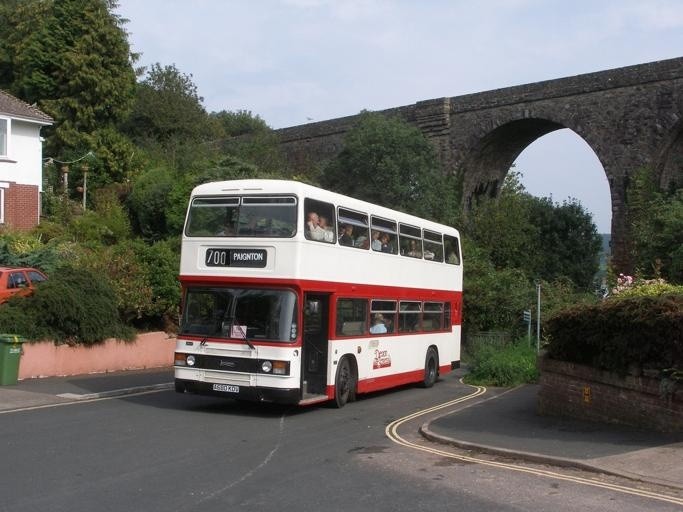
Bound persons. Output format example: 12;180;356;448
216;217;235;236
368;313;387;335
304;211;436;260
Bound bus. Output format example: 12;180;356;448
168;178;466;414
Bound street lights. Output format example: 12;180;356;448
533;278;541;356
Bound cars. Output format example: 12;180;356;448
0;266;48;303
